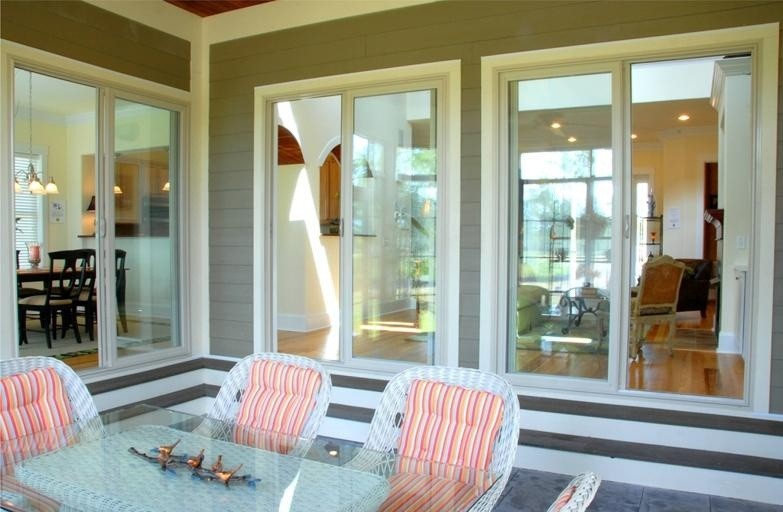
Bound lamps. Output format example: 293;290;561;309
14;70;59;197
85;195;95;236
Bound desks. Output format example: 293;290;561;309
0;403;503;512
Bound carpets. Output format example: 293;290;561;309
515;321;643;356
165;415;781;512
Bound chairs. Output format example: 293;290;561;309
0;354;113;512
517;284;549;335
596;253;714;360
15;247;130;349
